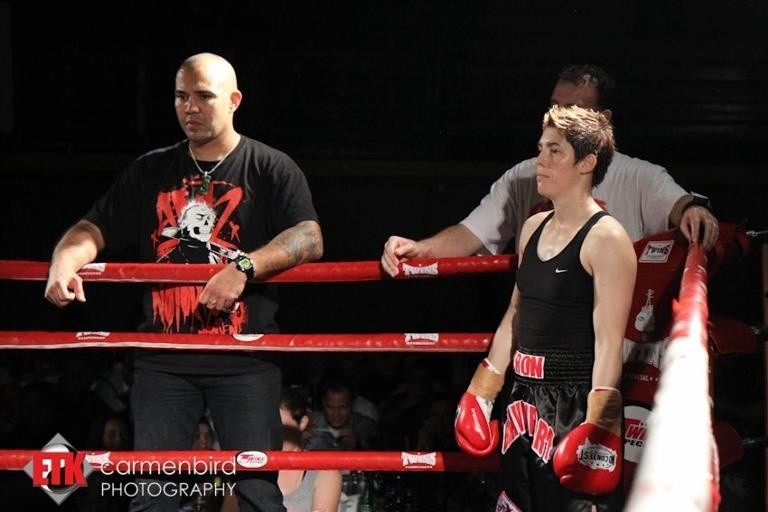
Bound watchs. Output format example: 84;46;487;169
233;254;256;282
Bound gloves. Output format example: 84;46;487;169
553;388;623;497
456;359;503;456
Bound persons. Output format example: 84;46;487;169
380;57;720;280
43;47;327;512
0;355;505;512
452;107;642;512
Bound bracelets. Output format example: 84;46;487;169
679;196;717;217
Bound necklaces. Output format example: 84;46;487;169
187;140;242;192
